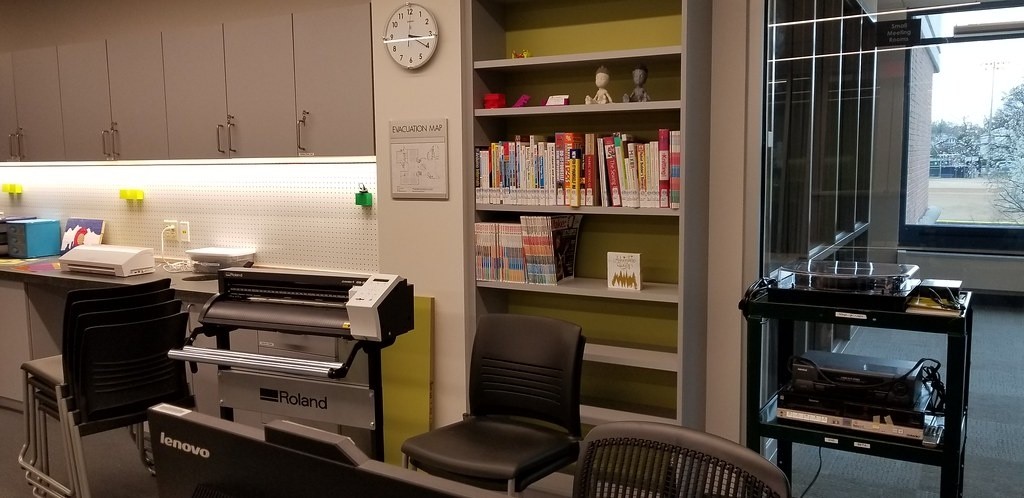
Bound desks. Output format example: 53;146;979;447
740;270;975;497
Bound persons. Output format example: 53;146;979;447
584;64;615;106
474;128;683;211
473;212;584;287
622;64;654;105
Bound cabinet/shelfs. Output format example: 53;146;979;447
456;1;716;497
0;1;376;167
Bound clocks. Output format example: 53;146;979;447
384;2;439;72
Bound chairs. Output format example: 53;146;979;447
16;274;198;498
397;312;794;498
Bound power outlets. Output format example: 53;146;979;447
163;219;179;241
